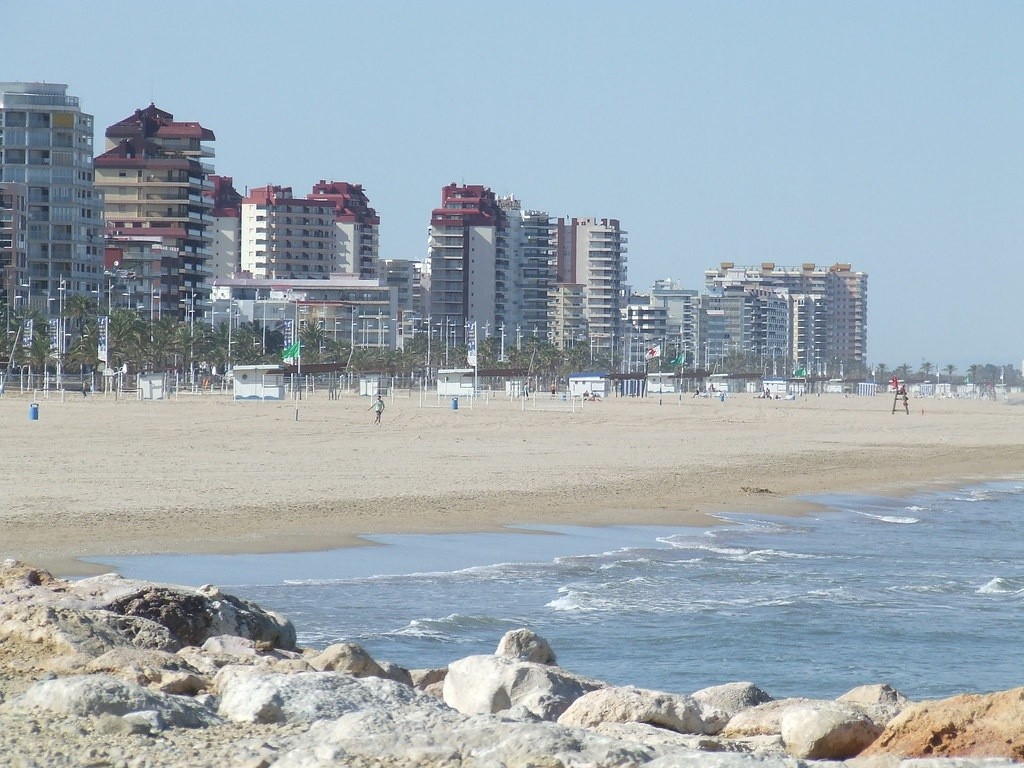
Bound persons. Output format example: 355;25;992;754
524;383;528;397
754;390;765;397
551;383;555;394
693;388;699;398
766;389;772;399
900;385;905;393
82;382;88;397
367;396;384;426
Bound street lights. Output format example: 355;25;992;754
1;275;845;416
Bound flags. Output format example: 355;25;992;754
282;341;301;357
670;353;683;365
645;346;660;360
795;368;804;375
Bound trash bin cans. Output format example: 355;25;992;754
720;394;724;402
29;403;39;420
451;398;458;409
